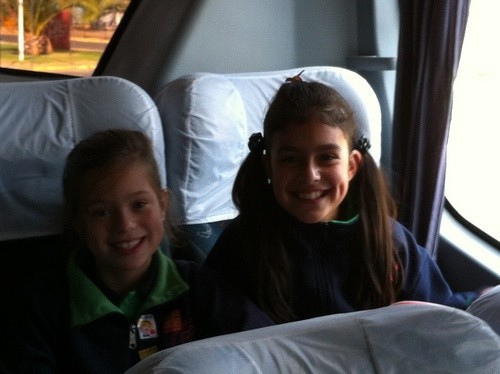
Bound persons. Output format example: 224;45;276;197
22;127;277;374
198;70;455;339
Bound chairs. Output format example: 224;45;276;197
0;76;170;276
152;66;382;285
123;301;499;374
